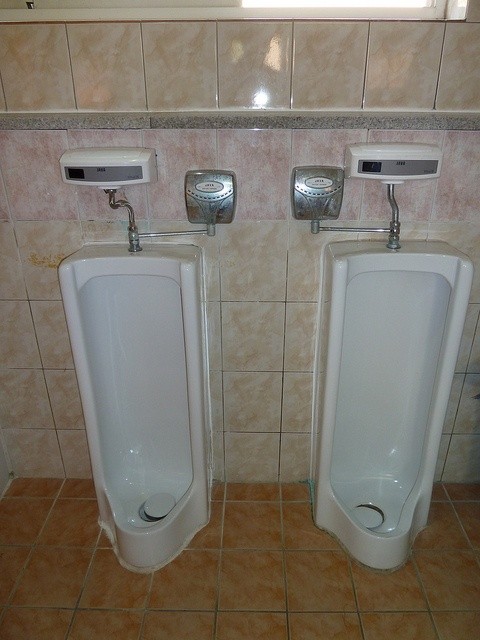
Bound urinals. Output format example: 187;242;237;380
59;243;211;570
319;240;474;568
